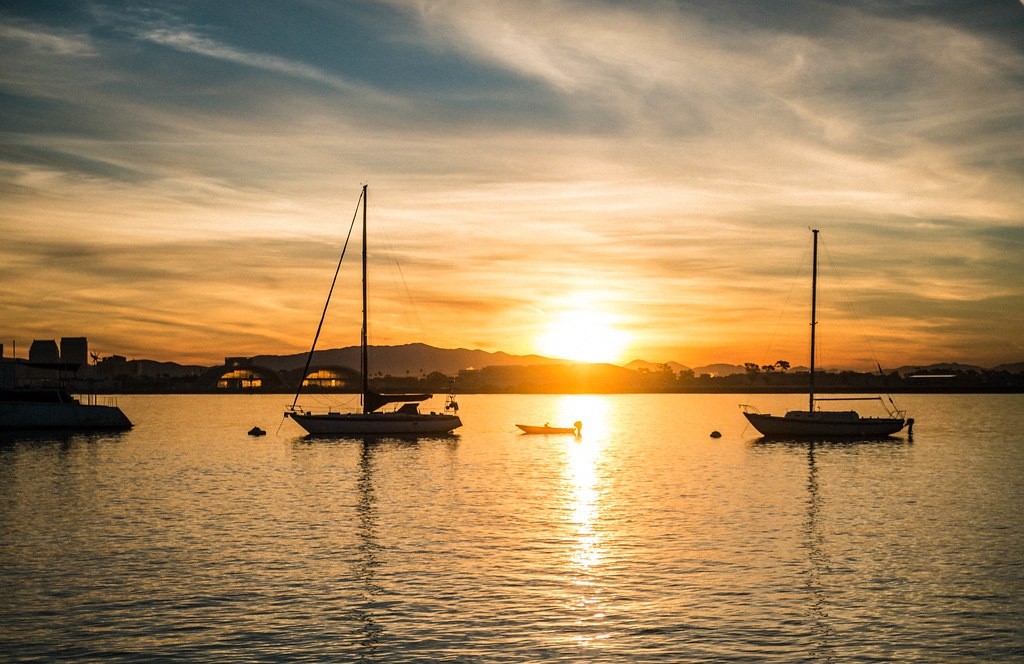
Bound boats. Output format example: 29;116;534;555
515;424;576;434
0;339;135;429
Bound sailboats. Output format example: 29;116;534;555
284;183;463;434
743;229;906;437
